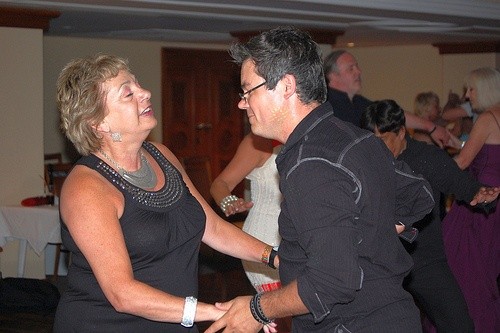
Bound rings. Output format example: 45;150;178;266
227;203;233;207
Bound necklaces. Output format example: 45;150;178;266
97;143;158;190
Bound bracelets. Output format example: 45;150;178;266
261;244;272;264
249;293;272;325
181;296;198;327
267;249;279;268
219;195;239;212
428;126;438;136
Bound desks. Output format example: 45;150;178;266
0;205;63;279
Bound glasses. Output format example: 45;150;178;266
239;82;267;103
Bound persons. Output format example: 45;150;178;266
204;27;434;333
362;100;500;333
415;86;479;216
52;53;283;332
324;50;462;150
444;67;500;333
209;127;285;298
